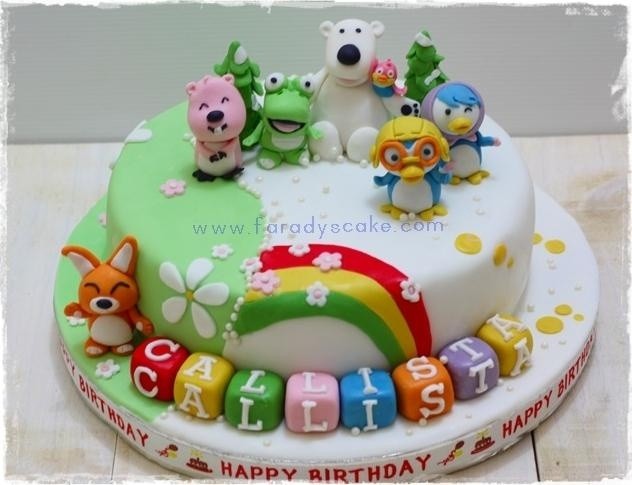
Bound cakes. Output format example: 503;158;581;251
62;18;537;433
